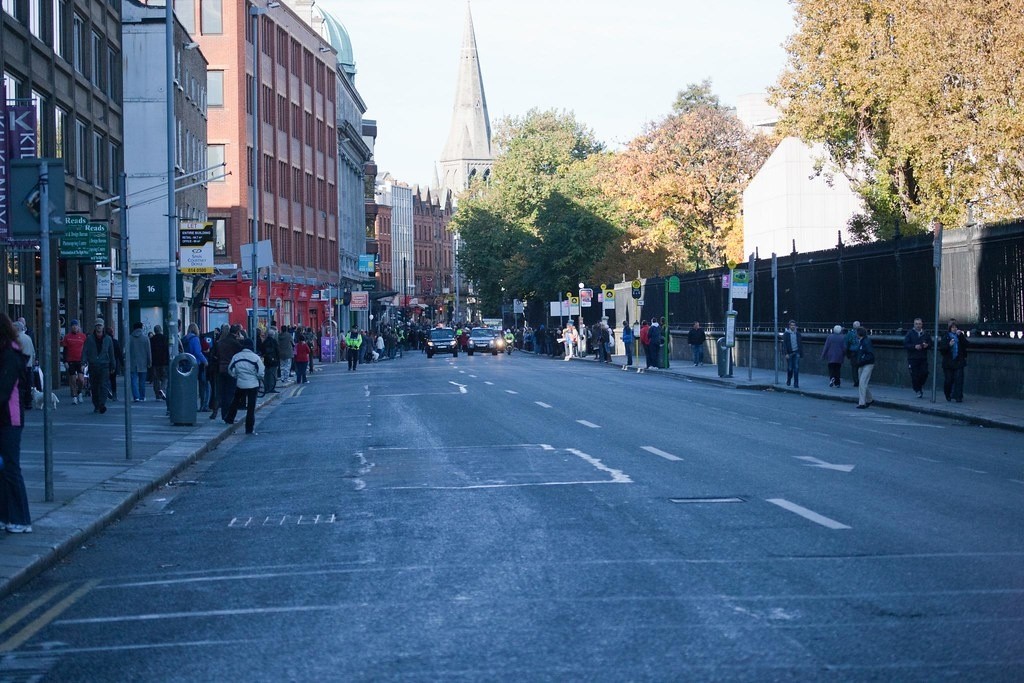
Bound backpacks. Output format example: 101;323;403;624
179;334;198;354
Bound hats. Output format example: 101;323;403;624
70;320;78;326
94;318;105;326
352;324;359;328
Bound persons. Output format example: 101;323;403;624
60;319;344;435
623;321;633;365
904;318;934;398
501;326;507;337
821;325;847;387
339;320;496;364
783;320;804;389
0;311;35;534
848;321;862;388
640;317;670;370
688;321;706;367
344;324;362;371
503;328;515;351
939;318;970;403
510;322;613;363
857;326;875;409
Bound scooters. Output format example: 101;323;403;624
504;338;514;355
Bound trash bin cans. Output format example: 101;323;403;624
717;337;734;378
658;336;669;368
167;353;199;427
578;334;587;357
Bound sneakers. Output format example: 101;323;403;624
0;522;32;533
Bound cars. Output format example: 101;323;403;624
493;331;505;353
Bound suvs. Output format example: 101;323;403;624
466;327;498;355
423;327;460;359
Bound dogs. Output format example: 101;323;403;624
31;386;59;410
371;351;379;360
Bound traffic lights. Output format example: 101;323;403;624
439;306;442;314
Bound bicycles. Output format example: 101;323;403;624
256;361;277;397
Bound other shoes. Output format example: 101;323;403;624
78;393;85;403
140;397;146;401
304;381;310;384
72;397;78;404
954;395;962;402
158;388;167;400
787;377;791;386
916;391;922;398
856;405;866;408
829;377;835;387
133;397;139;401
866;400;874;407
209;413;217;419
837;385;840;388
93;404;107;413
156;395;163;402
946;393;951;401
